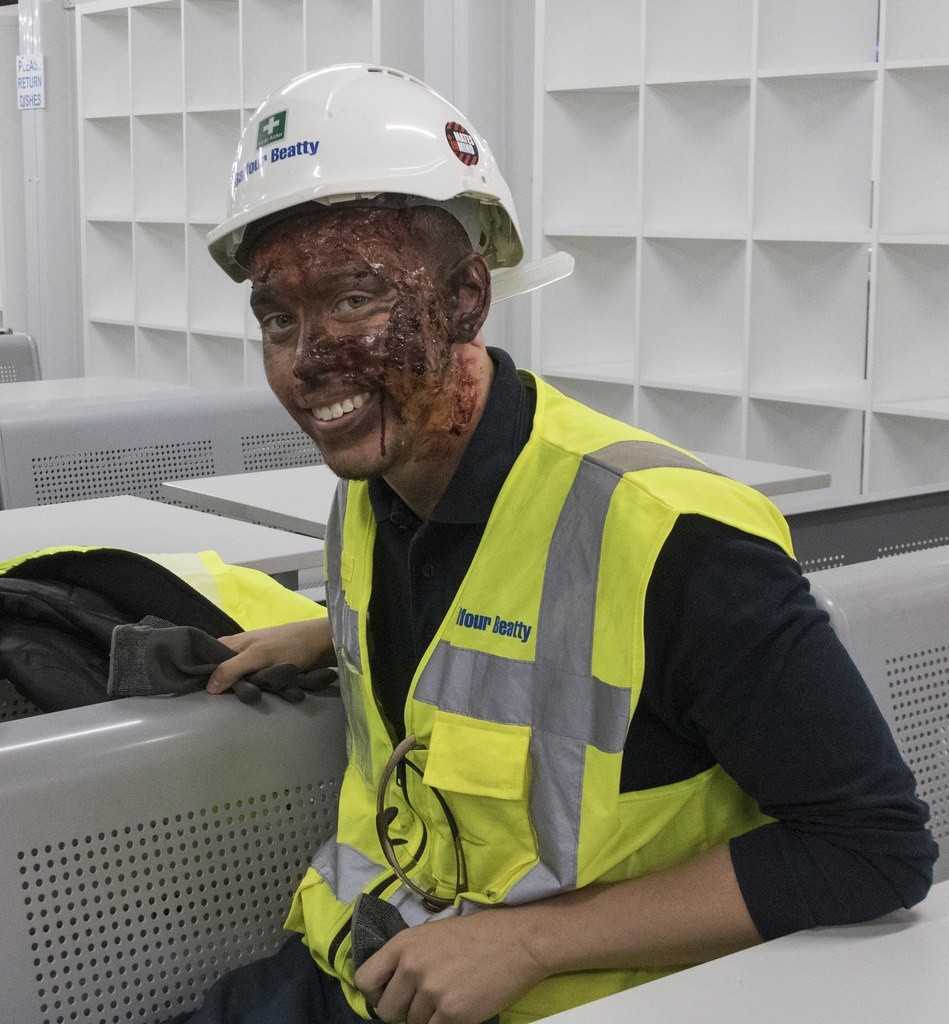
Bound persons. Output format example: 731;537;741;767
151;64;938;1023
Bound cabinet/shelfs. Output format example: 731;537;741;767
74;0;509;388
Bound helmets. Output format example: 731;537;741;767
205;63;525;283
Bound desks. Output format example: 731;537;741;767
162;452;832;539
0;375;190;402
531;879;948;1022
0;495;325;591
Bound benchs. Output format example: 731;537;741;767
0;332;949;1024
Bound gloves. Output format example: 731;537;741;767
350;893;499;1024
106;614;337;704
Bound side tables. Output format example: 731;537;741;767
534;0;949;496
